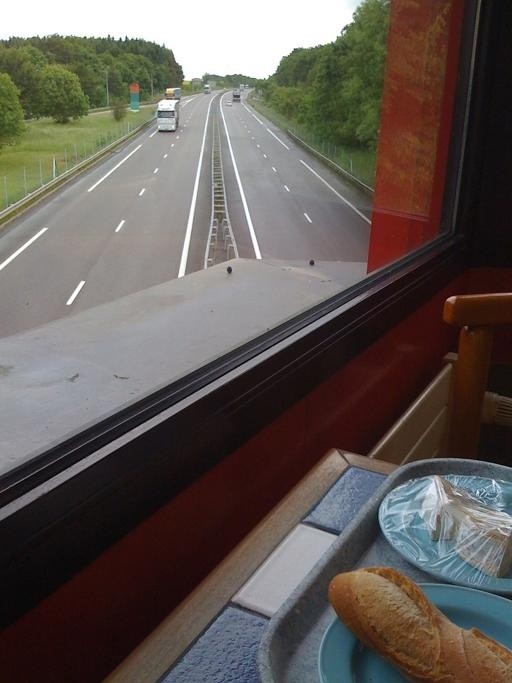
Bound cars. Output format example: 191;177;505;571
226;101;232;106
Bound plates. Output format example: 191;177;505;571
321;476;512;683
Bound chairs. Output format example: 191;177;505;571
442;293;512;460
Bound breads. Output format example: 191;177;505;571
422;473;501;541
328;567;512;683
454;513;510;578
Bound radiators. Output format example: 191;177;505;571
370;353;458;464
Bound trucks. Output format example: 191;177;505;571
233;84;249;102
156;100;179;132
203;84;211;94
165;88;181;100
